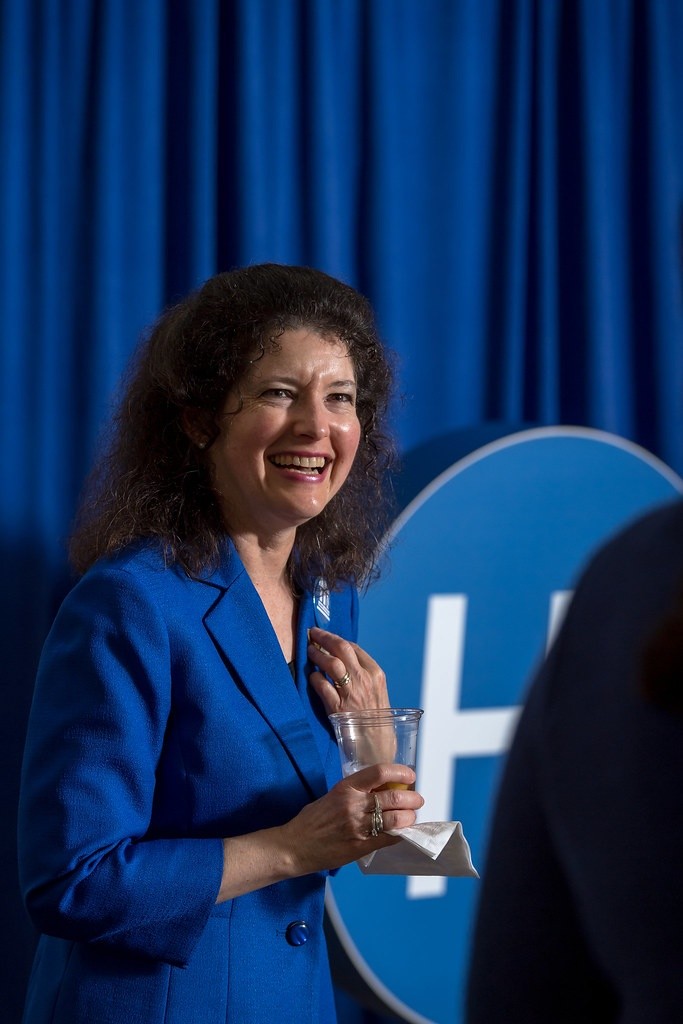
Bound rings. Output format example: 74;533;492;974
335;672;349;688
374;792;381;813
372;812;383;836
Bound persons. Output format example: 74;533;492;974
466;499;682;1024
17;265;426;1024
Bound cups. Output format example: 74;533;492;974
329;710;423;792
